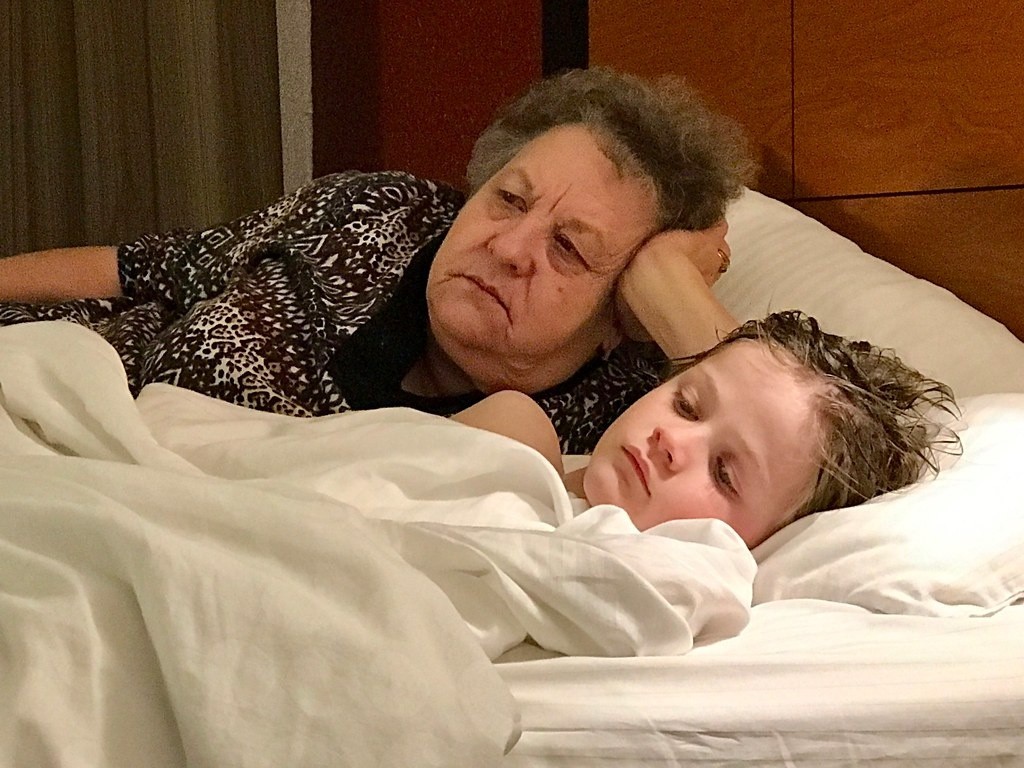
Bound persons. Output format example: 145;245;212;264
1;68;742;452
454;308;961;547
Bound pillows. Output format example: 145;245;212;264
716;182;1024;620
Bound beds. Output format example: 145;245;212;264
0;321;1024;768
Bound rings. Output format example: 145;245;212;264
717;248;730;274
